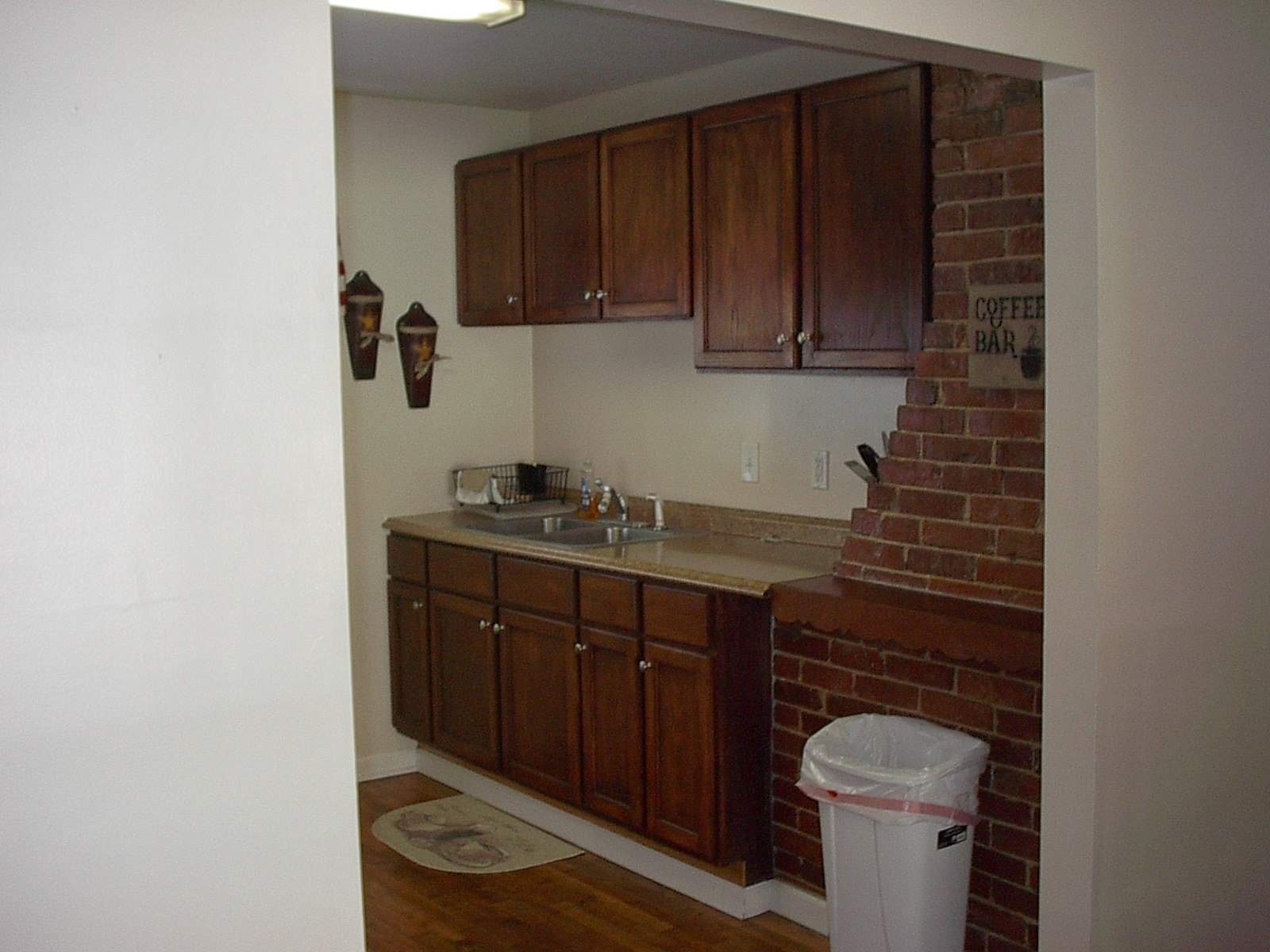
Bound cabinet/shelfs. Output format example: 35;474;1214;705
454;65;930;371
385;532;772;869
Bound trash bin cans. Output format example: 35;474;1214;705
804;713;990;952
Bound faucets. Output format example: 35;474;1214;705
594;479;630;522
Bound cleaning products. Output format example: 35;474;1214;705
576;458;600;521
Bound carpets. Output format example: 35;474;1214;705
373;794;583;874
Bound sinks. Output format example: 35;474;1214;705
459;515;596;538
519;523;677;550
